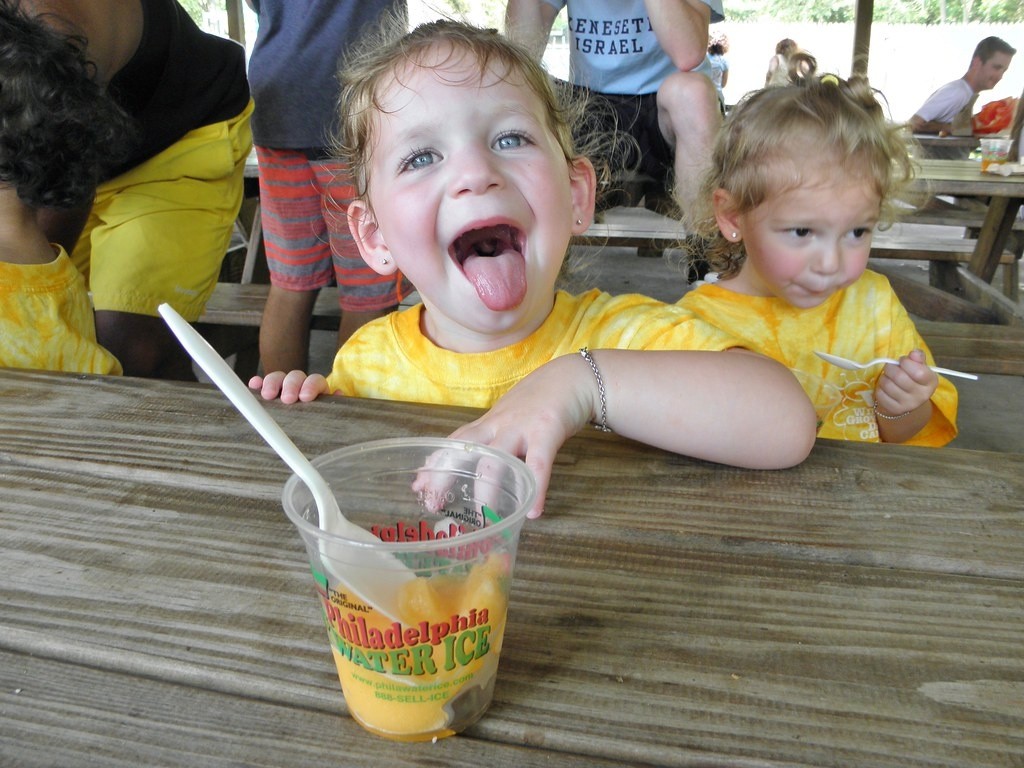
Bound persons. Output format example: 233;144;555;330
242;19;821;529
763;39;799;90
902;37;1024;259
0;0;255;377
674;74;960;447
224;0;409;383
505;0;725;290
707;39;729;114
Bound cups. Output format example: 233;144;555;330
279;437;537;741
979;139;1013;172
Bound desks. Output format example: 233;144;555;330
904;134;1011;158
0;367;1024;768
892;159;1024;323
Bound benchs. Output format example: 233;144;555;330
569;226;684;258
916;325;1024;376
199;282;341;333
880;211;1024;230
868;236;1016;264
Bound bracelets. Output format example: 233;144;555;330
871;398;911;421
579;346;612;433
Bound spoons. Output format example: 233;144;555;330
813;351;979;380
156;302;418;630
